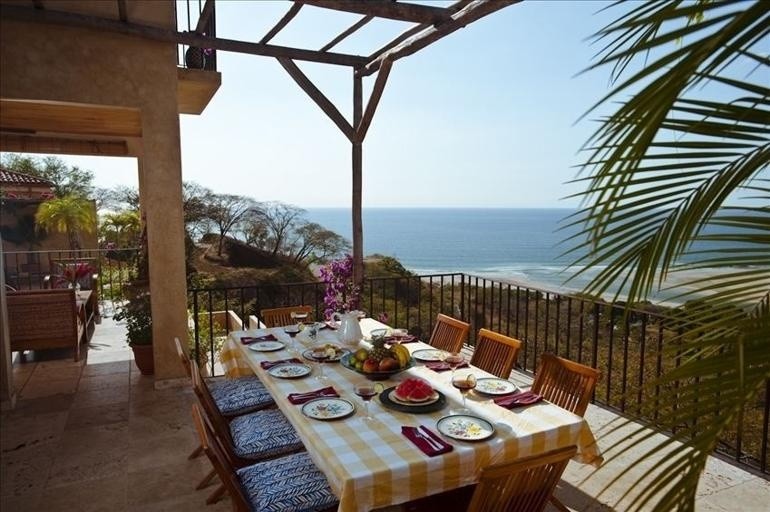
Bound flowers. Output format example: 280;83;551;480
317;255;365;323
49;263;98;291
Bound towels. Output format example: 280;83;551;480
326;322;340;331
287;386;338;405
260;358;304;370
384;334;419;345
425;360;469;372
400;424;455;459
239;333;278;344
493;390;545;410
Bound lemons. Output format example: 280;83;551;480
467;374;477;386
373;383;384;394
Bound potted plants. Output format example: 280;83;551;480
112;273;154;376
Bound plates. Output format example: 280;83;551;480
265;364;312;378
437;415;494;441
249;341;287;351
302;347;351;363
301;398;354;421
303;322;326;329
474;378;517;395
370;329;392;336
341;351;417;381
379;385;446;413
412;349;449;361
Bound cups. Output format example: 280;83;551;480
304;325;320;345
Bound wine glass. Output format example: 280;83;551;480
352;376;379;422
444;353;464;384
285;310;308;351
311;347;330;382
452;371;477;416
392;329;408;344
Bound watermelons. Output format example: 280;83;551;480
394;378;433;402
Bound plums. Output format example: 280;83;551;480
356;348;368;361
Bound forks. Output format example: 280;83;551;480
508;395;536;403
244;337;267;343
264;361;292;367
292;390;337;401
412;428;440;452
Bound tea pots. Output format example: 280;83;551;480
331;312;366;345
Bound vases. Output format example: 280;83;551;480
69;280;78;290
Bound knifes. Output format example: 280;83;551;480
417;426;445;450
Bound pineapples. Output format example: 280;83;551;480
368;346;392;362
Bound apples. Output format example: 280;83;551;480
363;358;379;372
379;357;398;371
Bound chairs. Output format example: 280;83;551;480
173;335;275;462
42;258;102;325
466;443;578;512
429;311;470;355
259;303;315;327
530;351;601;419
469;326;524;381
191;403;345;512
187;357;304;491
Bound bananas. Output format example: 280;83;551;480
390;342;410;368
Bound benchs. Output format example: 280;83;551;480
5;290;84;363
